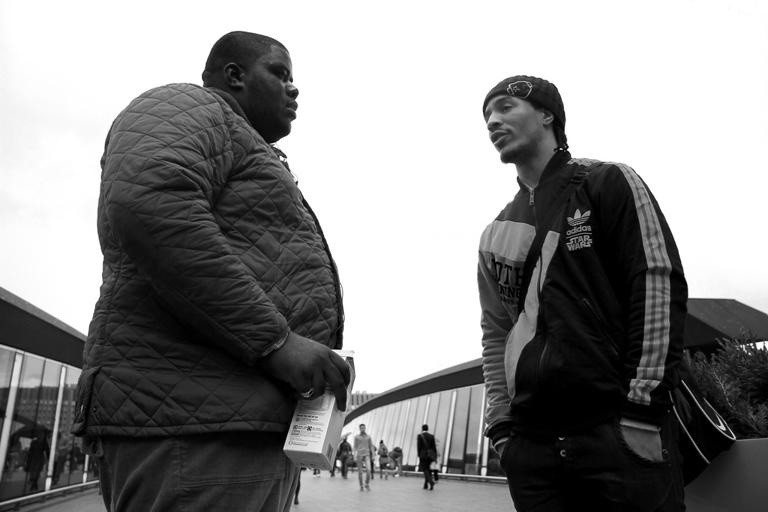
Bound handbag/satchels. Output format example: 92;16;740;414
661;374;738;486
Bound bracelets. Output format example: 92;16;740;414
261;332;290;357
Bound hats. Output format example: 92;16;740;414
482;75;566;129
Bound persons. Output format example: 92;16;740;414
3;427;100;491
313;423;402;492
477;74;689;512
430;435;442;484
294;481;301;504
417;425;437;490
70;31;350;512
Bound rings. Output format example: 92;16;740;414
301;387;313;398
